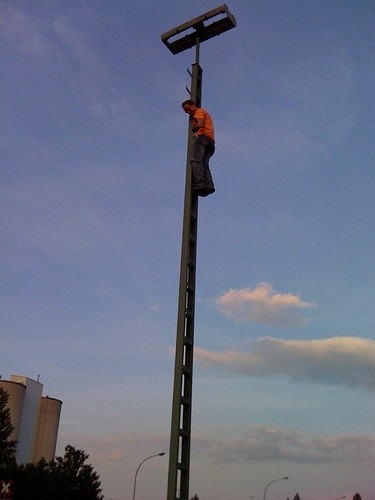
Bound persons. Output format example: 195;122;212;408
181;98;216;197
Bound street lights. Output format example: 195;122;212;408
133;452;166;500
263;476;289;500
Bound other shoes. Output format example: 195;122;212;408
194;187;215;196
